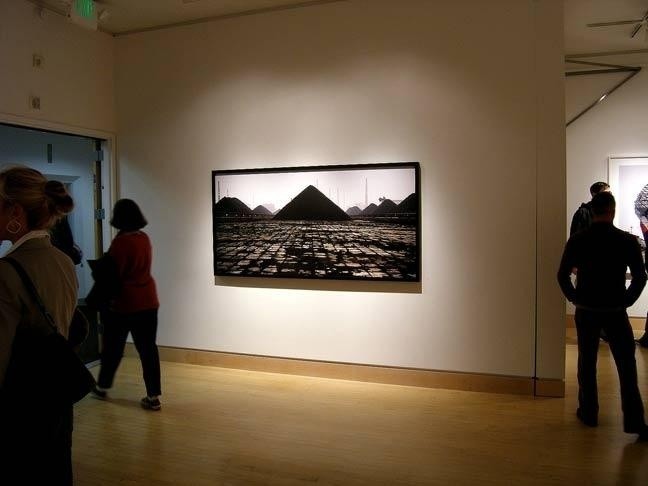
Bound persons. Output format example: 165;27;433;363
46;214;84;268
568;180;612;344
0;163;81;485
625;231;647;349
84;196;163;411
555;188;648;434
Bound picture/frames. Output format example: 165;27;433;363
211;162;422;283
608;154;648;274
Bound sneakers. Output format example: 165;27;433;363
140;396;162;411
91;384;107;400
575;406;648;438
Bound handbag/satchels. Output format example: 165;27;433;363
0;256;98;414
